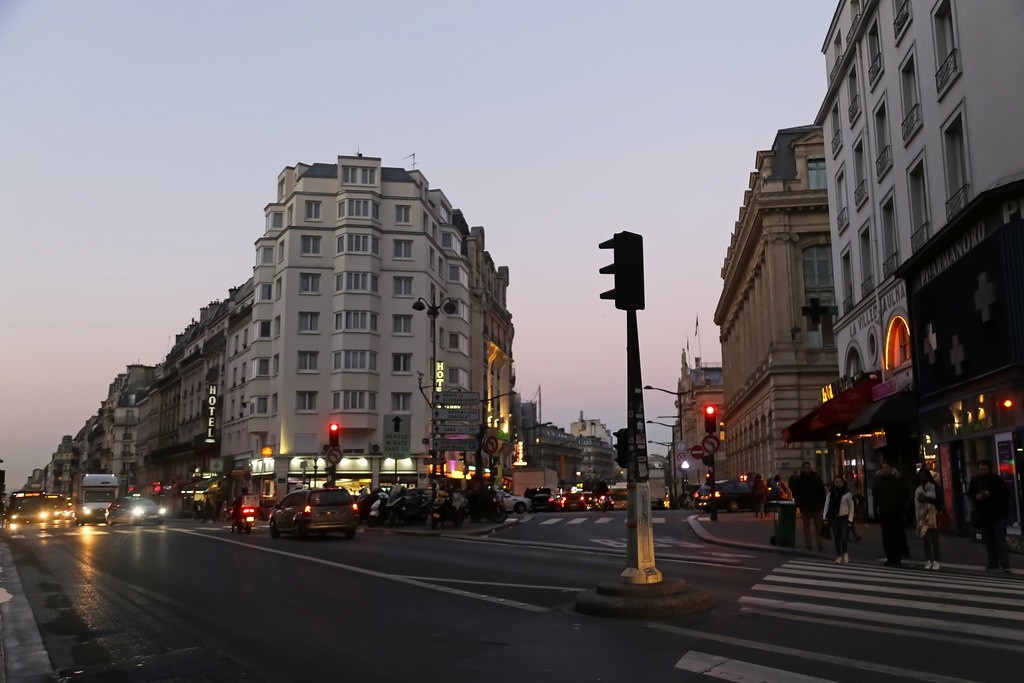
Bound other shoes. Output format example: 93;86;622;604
884;561;902;567
835;553;849;564
818;547;824;550
985;567;998;572
847;536;861;542
931;561;939;570
924;560;932;569
1003;569;1010;574
805;546;812;550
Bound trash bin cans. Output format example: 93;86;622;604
769;501;797;547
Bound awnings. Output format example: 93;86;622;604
781;378;881;442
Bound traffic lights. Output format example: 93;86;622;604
702;402;717;432
598;231;645;309
328;422;339;447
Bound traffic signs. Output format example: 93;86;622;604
433;392;481;450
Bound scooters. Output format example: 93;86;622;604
433;492;470;528
230;504;256;534
366;487;431;525
467;492;509;523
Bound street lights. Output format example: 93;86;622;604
644;383;707;510
411;297;457;489
476;391;516;490
525;422;553;476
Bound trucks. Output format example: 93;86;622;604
71;472;119;527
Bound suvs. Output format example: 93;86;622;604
267;486;359;541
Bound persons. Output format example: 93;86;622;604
201;488;251;533
752;460;944;571
967;459;1013;572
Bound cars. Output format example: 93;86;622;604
523;482;631;512
692;479;753;513
105;494;166;526
494;490;531;513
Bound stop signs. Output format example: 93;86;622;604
690;445;705;459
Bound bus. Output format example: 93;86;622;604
7;490;77;523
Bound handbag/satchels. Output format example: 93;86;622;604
820;520;832;539
936;509;950;530
